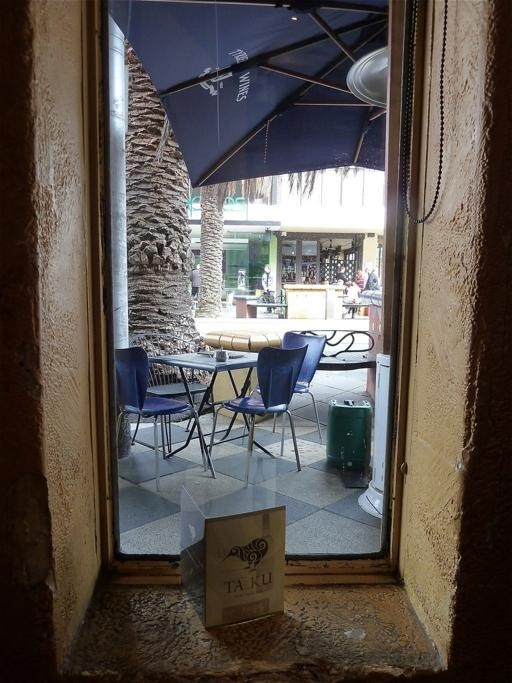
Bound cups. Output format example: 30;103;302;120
215;350;227;361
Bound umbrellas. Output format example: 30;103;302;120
106;1;390;189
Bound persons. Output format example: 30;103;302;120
190;261;201;296
335;264;378;292
261;263;276;313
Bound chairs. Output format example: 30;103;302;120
115;331;328;493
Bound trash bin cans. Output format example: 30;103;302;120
234;295;259;318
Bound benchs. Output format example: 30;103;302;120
247;303;288;318
